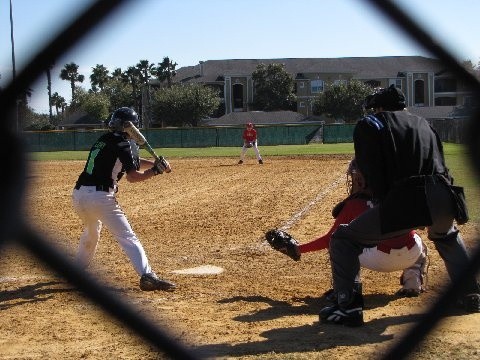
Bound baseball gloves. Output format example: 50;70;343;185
245;143;253;148
266;228;300;263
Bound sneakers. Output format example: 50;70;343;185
324;283;364;309
467;292;480;312
259;160;264;164
140;271;176;292
238;160;243;164
395;288;419;297
320;304;362;327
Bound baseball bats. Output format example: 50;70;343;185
123;120;171;173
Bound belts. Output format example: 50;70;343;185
74;185;110;192
377;238;416;254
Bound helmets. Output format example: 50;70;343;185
365;85;406;113
247;123;253;128
345;159;365;197
109;107;140;131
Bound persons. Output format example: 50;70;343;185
319;84;480;328
265;158;430;304
72;106;176;292
238;122;263;164
